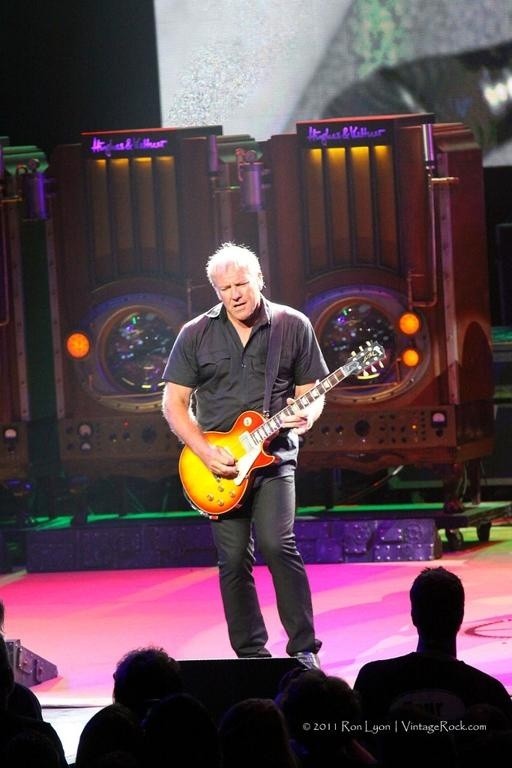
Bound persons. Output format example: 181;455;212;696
158;241;333;673
0;631;511;768
352;565;510;712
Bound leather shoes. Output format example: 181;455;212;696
297;652;320;670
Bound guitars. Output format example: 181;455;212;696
177;339;387;515
